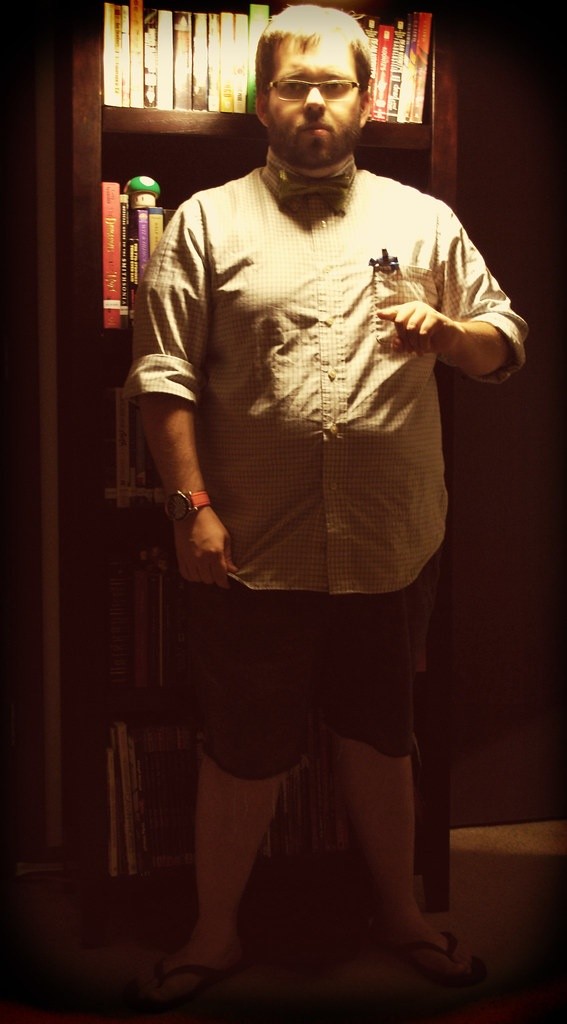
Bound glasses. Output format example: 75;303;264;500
264;76;362;102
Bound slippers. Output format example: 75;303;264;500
135;943;252;1009
390;925;487;989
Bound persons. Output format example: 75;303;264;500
125;5;527;1015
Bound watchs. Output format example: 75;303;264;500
163;489;212;525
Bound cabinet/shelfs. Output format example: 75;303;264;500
36;0;454;923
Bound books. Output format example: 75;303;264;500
102;0;435;126
112;382;174;509
102;177;182;333
105;529;364;882
